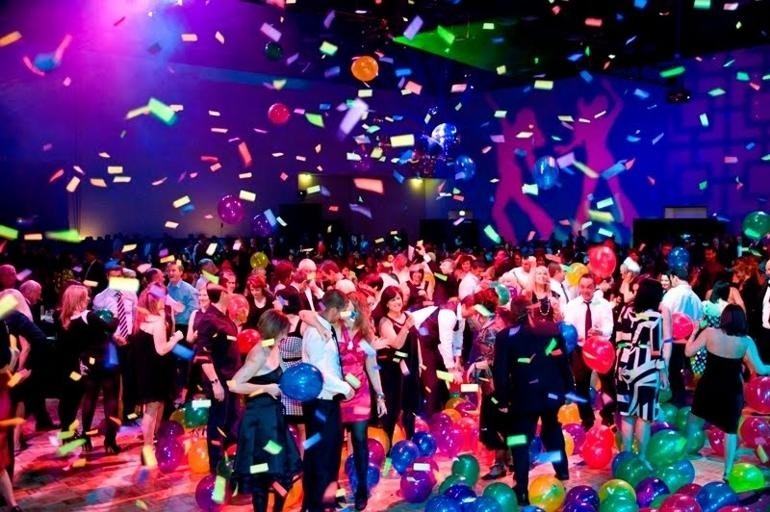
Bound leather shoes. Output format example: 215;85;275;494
33;419;63;432
481;470;507;481
515;487;528;506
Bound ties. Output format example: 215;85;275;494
115;292;130;341
582;300;595;340
453;320;459;331
330;323;346;383
560;283;569;302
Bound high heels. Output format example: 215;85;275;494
79;432;95;453
104;435;122;454
721;472;731;484
355;485;368;511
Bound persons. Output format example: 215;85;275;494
1;227;770;509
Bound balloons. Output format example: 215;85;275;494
15;214;35;228
431;122;462;151
250;214;271;237
267;103;290;126
264;42;284;62
218;195;244;225
455;154;478;181
351;57;379;82
531;154;561;191
742;211;770;241
